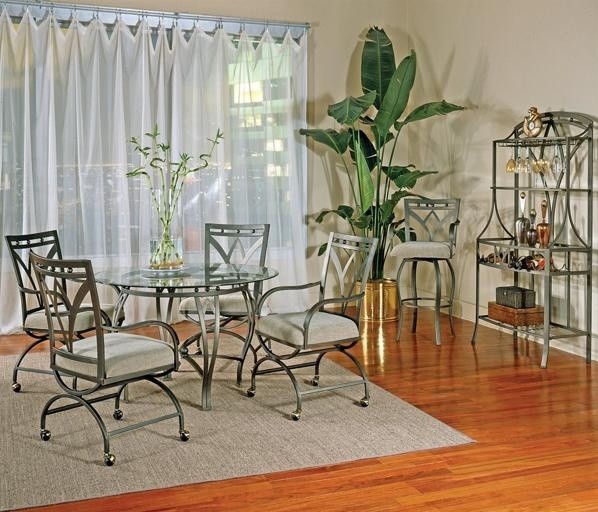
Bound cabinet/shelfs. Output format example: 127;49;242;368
470;110;594;369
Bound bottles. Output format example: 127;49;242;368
477;251;553;272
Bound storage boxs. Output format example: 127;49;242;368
496;285;535;308
487;301;543;327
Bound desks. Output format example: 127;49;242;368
69;264;277;409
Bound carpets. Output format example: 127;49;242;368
1;350;476;512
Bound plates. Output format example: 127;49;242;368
140;266;190;276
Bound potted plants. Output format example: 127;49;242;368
293;25;463;325
120;124;221;269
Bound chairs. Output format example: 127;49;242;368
6;229;123;394
248;231;382;422
178;224;271;386
390;196;461;346
29;250;189;467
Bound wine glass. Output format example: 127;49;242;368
502;139;568;176
